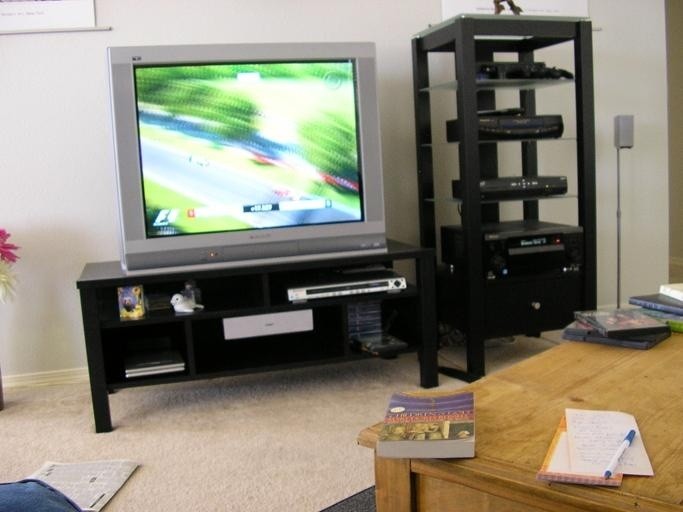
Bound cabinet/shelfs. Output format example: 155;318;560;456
412;18;598;384
75;238;439;433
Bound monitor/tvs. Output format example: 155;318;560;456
107;42;389;277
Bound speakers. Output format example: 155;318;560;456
614;114;634;149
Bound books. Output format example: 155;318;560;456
628;291;683;316
632;307;682;334
124;349;184;373
374;391;476;460
534;416;624;486
124;365;184;378
659;282;683;302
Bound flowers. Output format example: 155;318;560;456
0;227;22;305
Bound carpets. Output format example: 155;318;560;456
315;483;375;512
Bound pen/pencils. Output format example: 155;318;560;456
604;431;635;480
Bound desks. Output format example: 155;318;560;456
357;331;683;512
